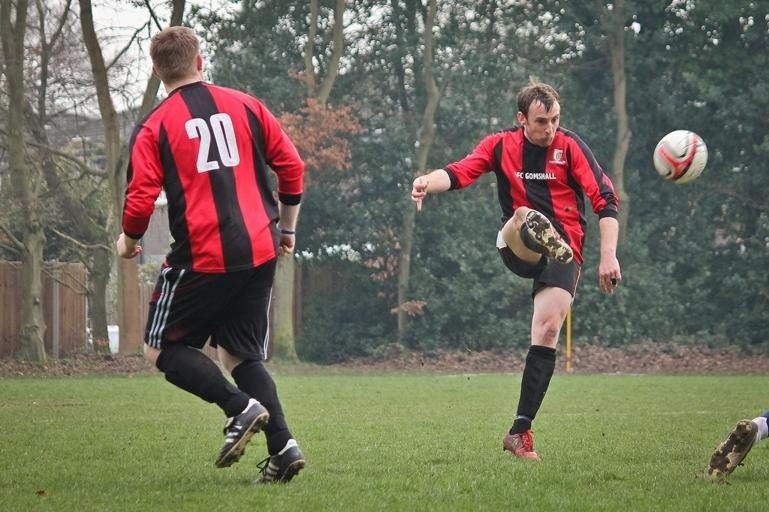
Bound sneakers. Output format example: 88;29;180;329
525;210;573;265
503;429;538;460
255;439;305;484
708;419;758;481
215;397;270;468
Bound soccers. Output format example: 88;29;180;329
653;130;708;183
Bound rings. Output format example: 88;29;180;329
611;278;616;285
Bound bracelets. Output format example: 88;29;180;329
277;227;295;235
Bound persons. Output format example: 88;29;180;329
115;26;310;490
706;411;769;478
411;85;630;466
